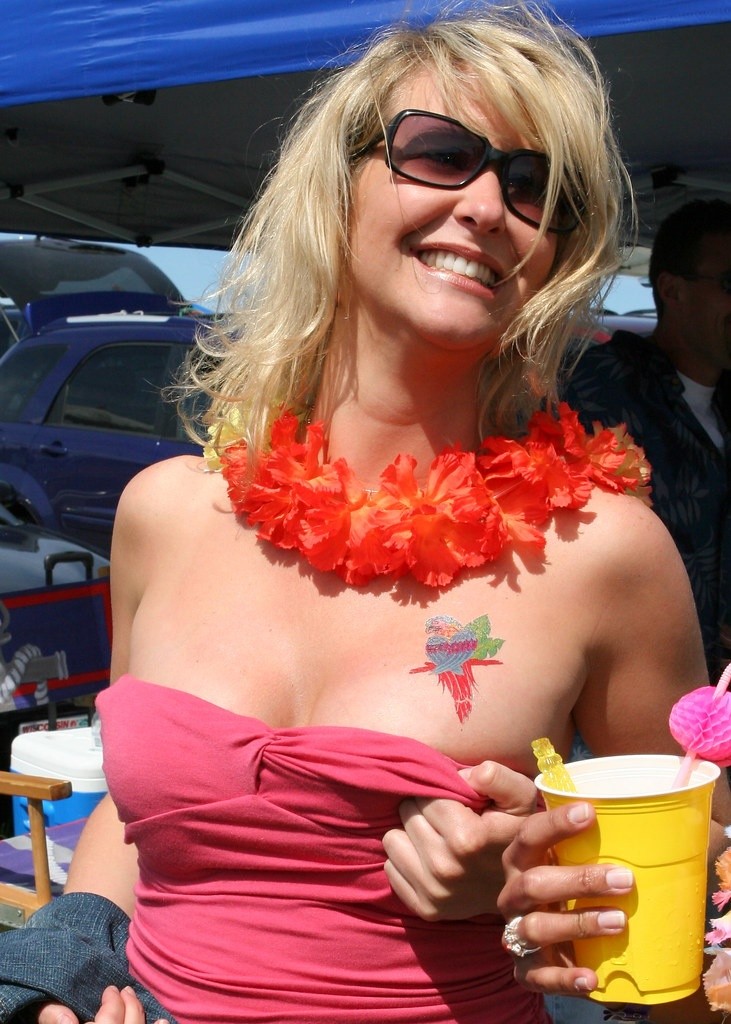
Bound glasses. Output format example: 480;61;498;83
348;109;588;276
677;270;731;293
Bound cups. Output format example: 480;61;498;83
535;755;721;1006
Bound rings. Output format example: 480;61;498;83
503;915;541;959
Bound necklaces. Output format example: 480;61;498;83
221;409;654;588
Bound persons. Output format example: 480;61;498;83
495;661;731;1023
37;1;731;1024
558;197;730;686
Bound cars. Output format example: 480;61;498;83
0;233;238;803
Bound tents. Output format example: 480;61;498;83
0;1;731;247
